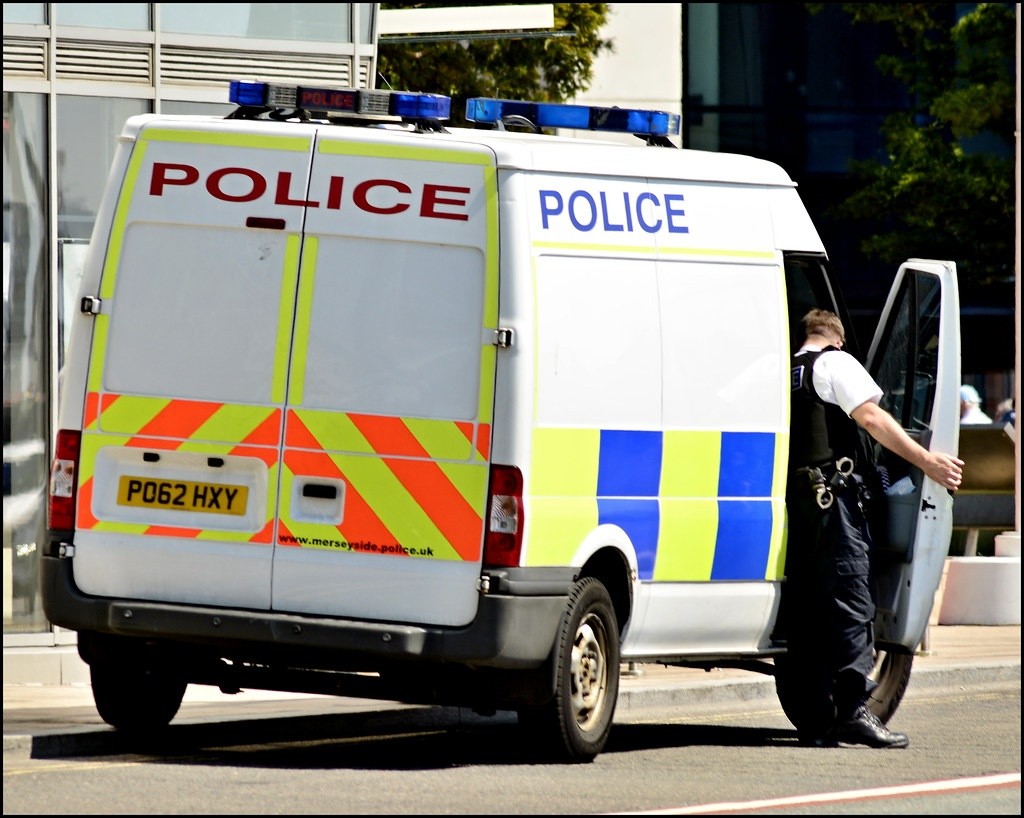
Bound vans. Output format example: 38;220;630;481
42;72;962;762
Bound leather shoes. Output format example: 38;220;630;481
834;703;909;749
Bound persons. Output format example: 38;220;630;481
995;397;1018;429
959;385;994;424
789;309;965;752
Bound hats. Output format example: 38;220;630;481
961;385;980;403
